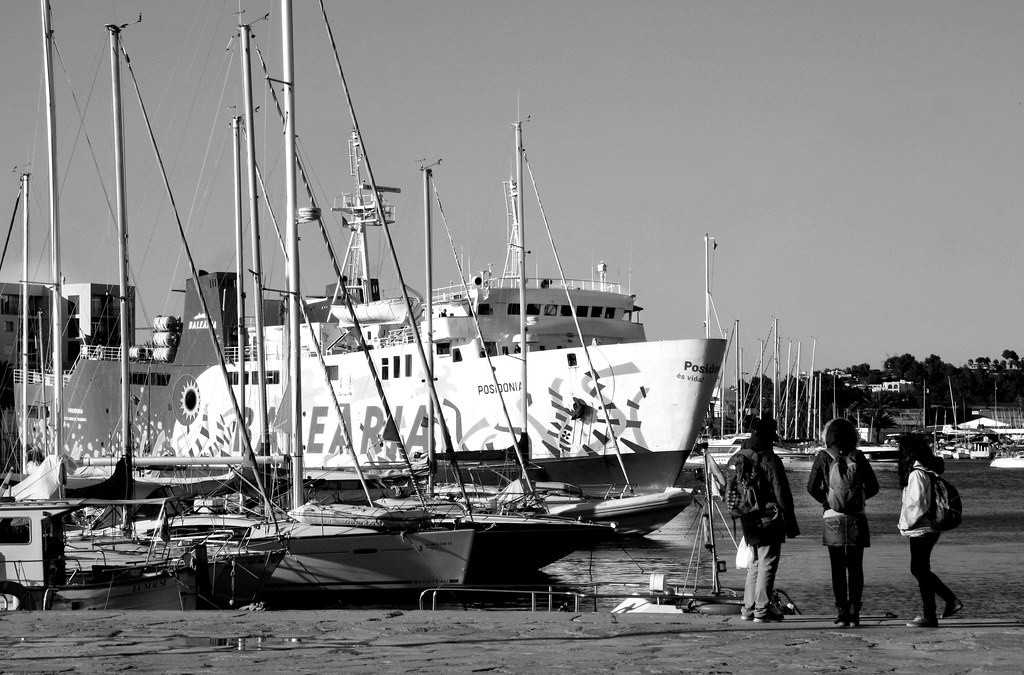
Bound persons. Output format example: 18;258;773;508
808;419;878;626
899;434;962;627
724;419;801;623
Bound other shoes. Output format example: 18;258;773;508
906;616;938;628
940;597;962;618
835;613;860;628
740;615;754;621
752;613;784;623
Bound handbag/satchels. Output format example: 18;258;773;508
735;534;754;571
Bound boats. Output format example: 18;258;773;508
953;447;971;459
970;441;995;459
990;451;1024;469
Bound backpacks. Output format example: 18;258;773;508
911;466;962;531
725;448;768;539
822;446;866;513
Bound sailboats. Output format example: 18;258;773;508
154;115;726;498
1;0;956;608
611;452;800;619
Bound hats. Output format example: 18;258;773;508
751;415;780;442
